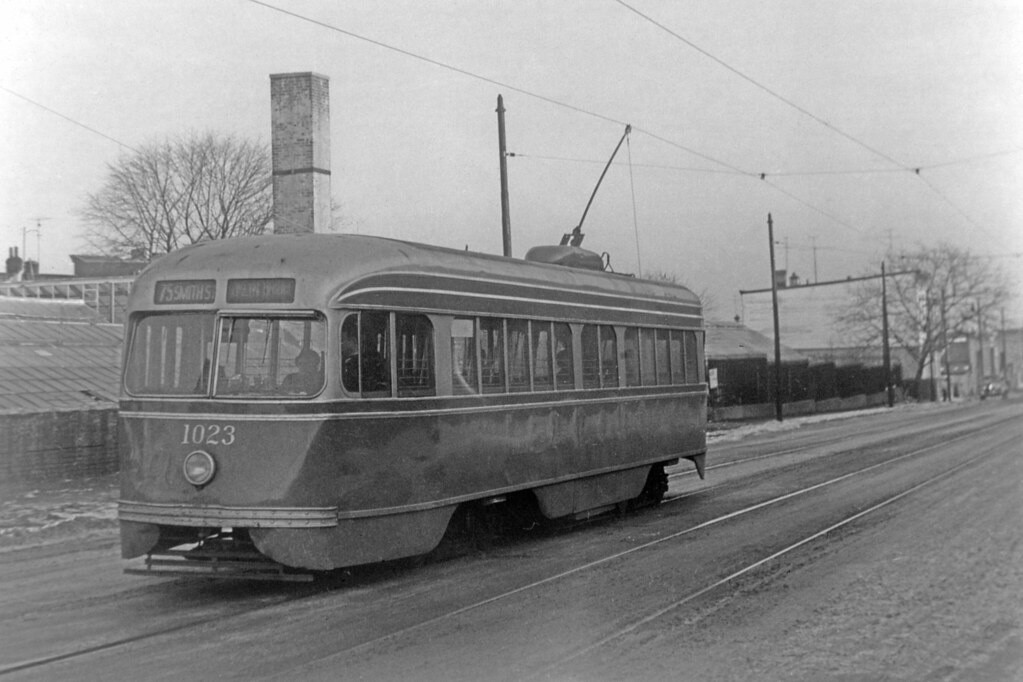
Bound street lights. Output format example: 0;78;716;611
942;302;975;402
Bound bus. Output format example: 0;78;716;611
116;233;709;582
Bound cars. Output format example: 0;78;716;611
971;375;1011;400
